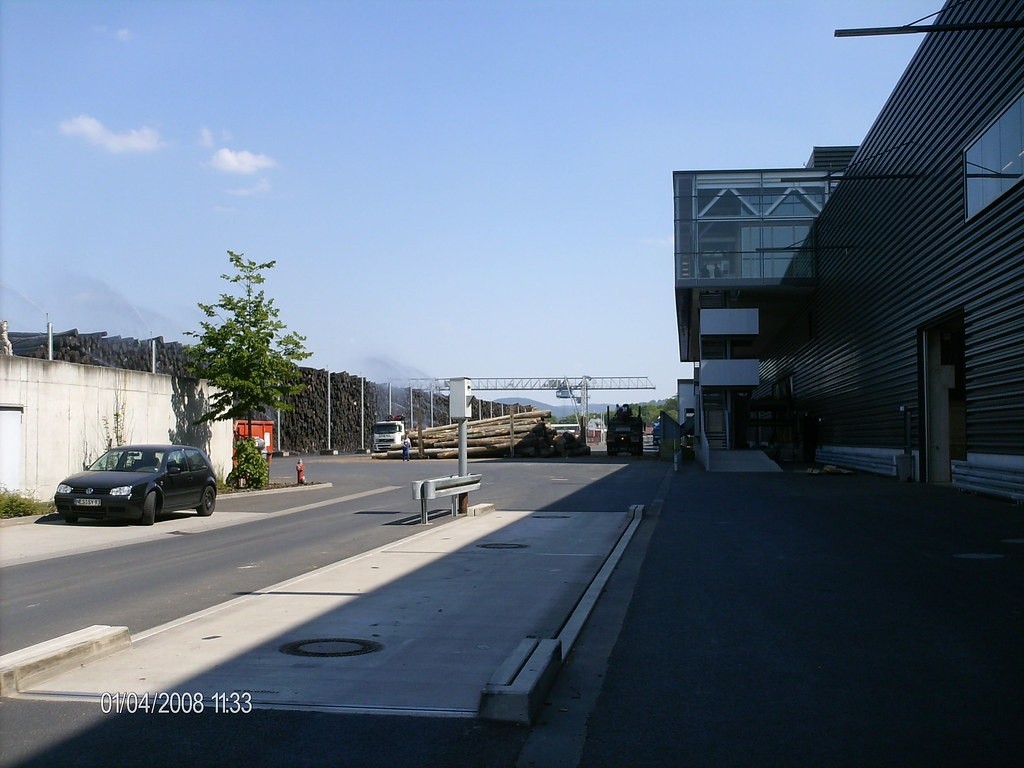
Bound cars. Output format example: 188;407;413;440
53;443;218;525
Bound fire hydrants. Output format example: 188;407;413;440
295;459;305;485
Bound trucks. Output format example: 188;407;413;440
232;418;277;467
602;405;646;456
370;421;407;453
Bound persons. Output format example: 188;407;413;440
402;434;411;461
